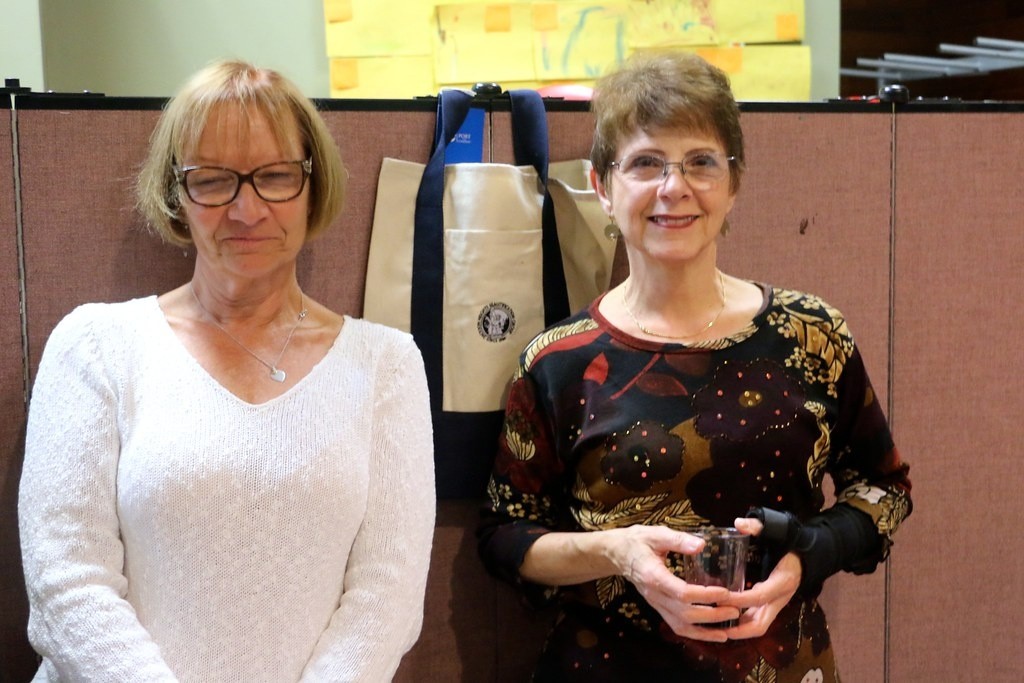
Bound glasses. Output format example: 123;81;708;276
172;155;312;207
603;155;738;183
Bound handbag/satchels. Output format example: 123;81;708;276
360;90;616;501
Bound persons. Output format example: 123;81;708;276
17;59;436;683
474;56;914;683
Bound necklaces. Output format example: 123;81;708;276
190;281;308;383
622;267;725;339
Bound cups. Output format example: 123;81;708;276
683;527;750;631
